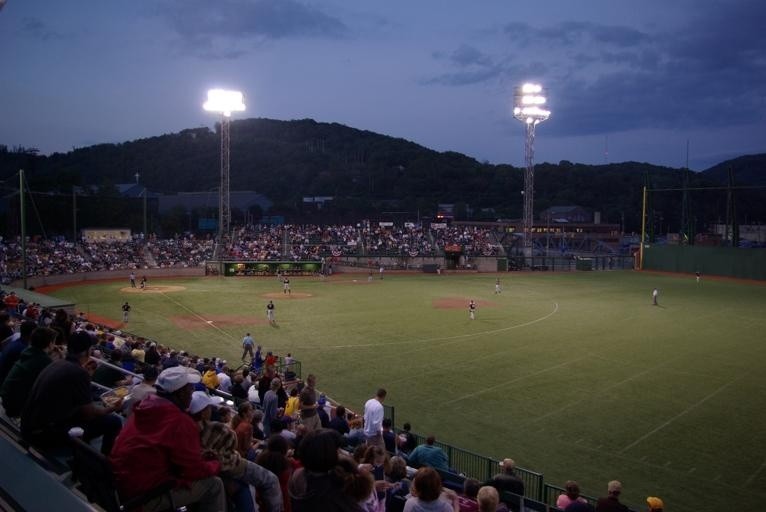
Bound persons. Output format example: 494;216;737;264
1;295;524;511
1;220;505;293
556;480;630;511
646;496;665;511
694;271;705;286
652;288;658;305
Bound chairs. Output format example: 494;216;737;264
1;302;590;511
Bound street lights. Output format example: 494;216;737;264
509;81;554;247
200;87;247;236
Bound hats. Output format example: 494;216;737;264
646;496;663;510
607;480;621;492
154;367;201;393
499;458;515;470
186;390;224;415
68;328;98;352
